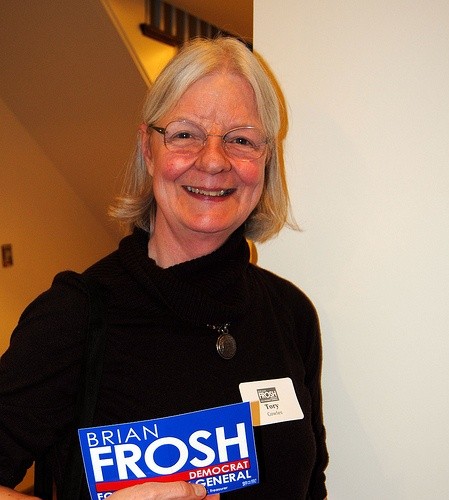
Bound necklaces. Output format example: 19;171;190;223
206;324;236;359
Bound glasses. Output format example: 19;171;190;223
150;121;273;163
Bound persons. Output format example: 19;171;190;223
0;38;329;500
